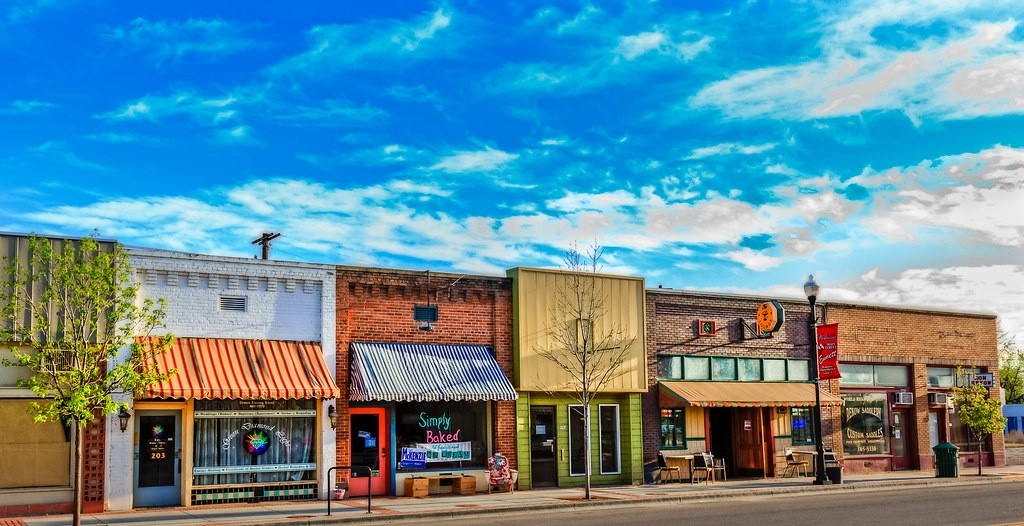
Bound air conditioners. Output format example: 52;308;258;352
37;348;79;374
928;393;947;404
894;392;913;405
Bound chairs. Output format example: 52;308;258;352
485;468;518;495
782;447;809;478
692;452;727;486
655;451;681;484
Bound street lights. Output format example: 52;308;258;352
803;275;832;485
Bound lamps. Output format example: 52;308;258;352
117;406;131;433
328;405;339;431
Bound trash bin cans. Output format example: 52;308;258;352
826;467;844;484
932;442;960;478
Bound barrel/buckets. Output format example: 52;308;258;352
826;466;844;484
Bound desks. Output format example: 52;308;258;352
792;451;818;477
666;454;715;483
422;473;463;479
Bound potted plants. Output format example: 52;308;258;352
404;474;429;498
451;474;476;495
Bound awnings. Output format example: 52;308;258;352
658;379;843;409
349;341;517;402
132;336;341;400
